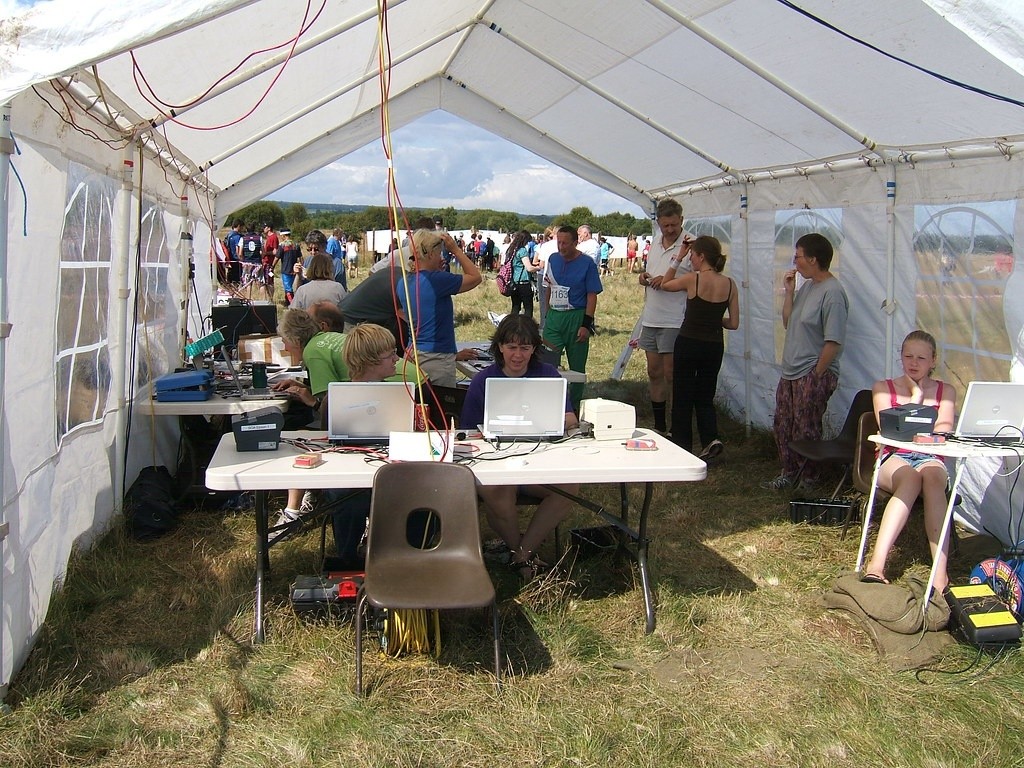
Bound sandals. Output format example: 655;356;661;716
508;553;542;580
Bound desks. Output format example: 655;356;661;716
453;357;588;386
215;301;278;359
847;433;1024;609
136;349;307;504
200;419;717;651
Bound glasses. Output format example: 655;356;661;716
307;248;320;252
380;348;397;359
794;254;808;259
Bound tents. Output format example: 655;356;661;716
0;0;1024;684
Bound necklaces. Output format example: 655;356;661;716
701;268;717;273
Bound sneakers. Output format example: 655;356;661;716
698;440;723;461
760;474;793;491
300;492;319;515
268;508;303;541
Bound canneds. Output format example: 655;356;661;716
414;403;430;432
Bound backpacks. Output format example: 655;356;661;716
496;246;524;297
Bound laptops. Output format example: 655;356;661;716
328;381;416;445
935;381;1024;442
477;378;568;442
221;344;290;400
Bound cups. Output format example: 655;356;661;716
302;268;308;278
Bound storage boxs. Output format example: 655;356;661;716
943;582;1022;653
880;401;939;439
292;570;371;627
788;489;863;524
568;522;654;568
155;365;217;402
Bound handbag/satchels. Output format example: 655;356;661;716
124;466;179;538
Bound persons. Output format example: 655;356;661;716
396;219;483;389
639;198;698;439
342;237;413;353
541;227;603;423
865;329;955;595
757;233;850;498
497;223;557;317
460;314;579;430
441;232;502;271
224;218;360;306
576;225;649;272
660;234;739;462
373;231;411;264
267;301;434;543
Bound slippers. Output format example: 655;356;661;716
860;574;886;583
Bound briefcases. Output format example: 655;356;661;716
157;369;217;401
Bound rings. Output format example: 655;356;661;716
297;388;299;392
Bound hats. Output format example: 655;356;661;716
261;221;274;228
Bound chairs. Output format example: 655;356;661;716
342;459;515;697
838;410;967;559
787;383;875;499
514;484;591;564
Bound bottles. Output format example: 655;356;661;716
252;363;267;388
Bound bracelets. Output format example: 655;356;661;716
671;259;681;269
313;398;322;411
582;314;595;336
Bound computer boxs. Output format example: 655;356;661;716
212;304;277;361
